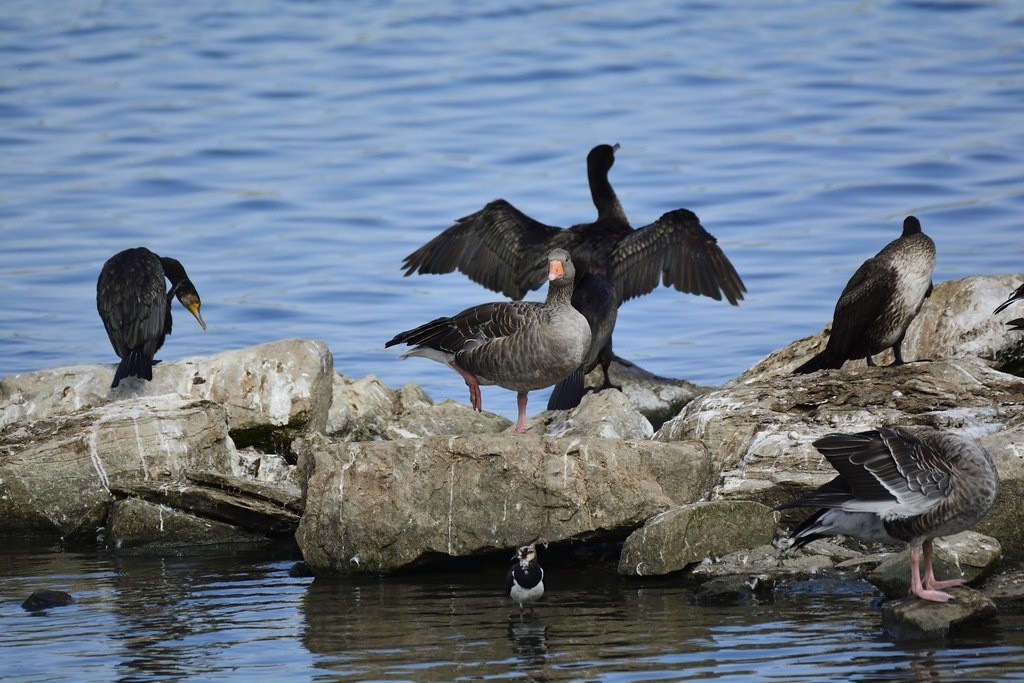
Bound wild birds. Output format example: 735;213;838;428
382;248;592;439
766;426;1000;603
792;216;937;376
399;143;748;411
94;246;207;388
505;532;545;615
